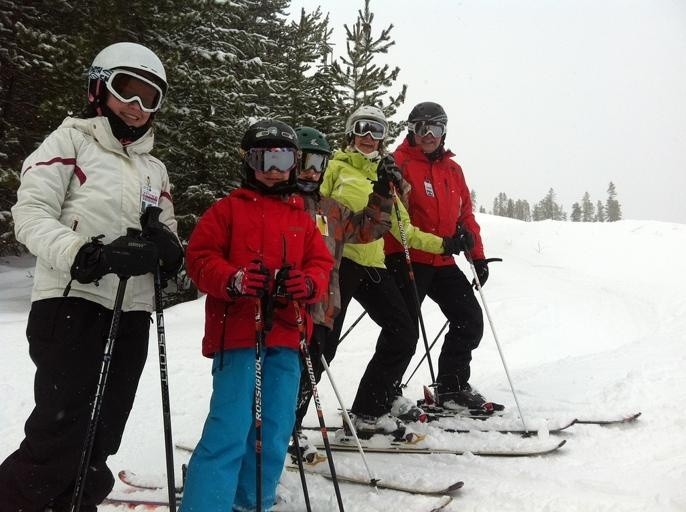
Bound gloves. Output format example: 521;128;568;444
472;259;488;290
441;228;474;256
367;155;403;199
64;224;184;299
286;270;314;301
226;263;270;299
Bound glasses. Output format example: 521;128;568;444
408;122;447;138
87;66;166;113
241;147;297;175
345;120;387;140
302;150;329;173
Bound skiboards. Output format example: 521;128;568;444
105;463;453;512
93;385;643;512
176;382;568;495
393;377;644;434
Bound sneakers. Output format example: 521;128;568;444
342;410;416;443
289;431;318;462
388;391;427;423
433;385;505;415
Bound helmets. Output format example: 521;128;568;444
408;101;448;126
87;42;169;104
345;106;389;136
241;118;333;155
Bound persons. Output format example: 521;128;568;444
389;102;504;418
176;120;336;512
1;40;184;512
292;125;405;466
291;105;474;465
